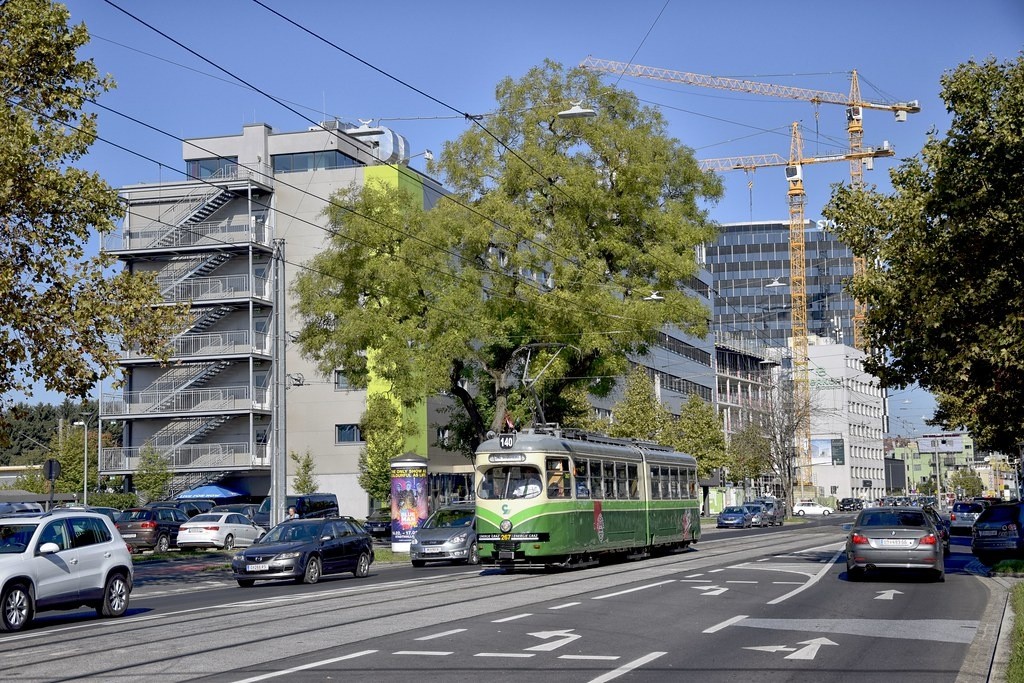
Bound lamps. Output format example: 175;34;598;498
643;291;666;301
901;398;913;404
759;357;776;364
558;97;598;118
766;277;788;288
920;415;927;419
396;148;433;164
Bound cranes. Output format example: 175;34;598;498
689;120;898;501
578;54;921;355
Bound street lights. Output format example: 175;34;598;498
73;419;88;506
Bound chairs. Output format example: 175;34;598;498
867;514;883;526
41;525;57;542
727;508;733;512
145;512;153;519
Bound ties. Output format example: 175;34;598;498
523;480;529;497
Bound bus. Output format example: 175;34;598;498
472;422;702;573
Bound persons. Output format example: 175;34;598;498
894;501;897;506
286;507;299;519
902;500;917;506
513;468;541;498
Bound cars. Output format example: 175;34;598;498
409;501;481;568
176;511;267;551
48;505;123;529
841;505;947;583
793;502;835;517
741;502;770;529
209;504;260;522
716;505;753;528
230;515;376;588
0;502;44;516
837;490;1004;554
970;501;1024;567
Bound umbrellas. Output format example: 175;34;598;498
175;484;250;498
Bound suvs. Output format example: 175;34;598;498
0;508;135;634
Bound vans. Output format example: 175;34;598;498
754;497;784;527
114;506;192;555
141;500;218;517
251;493;339;533
